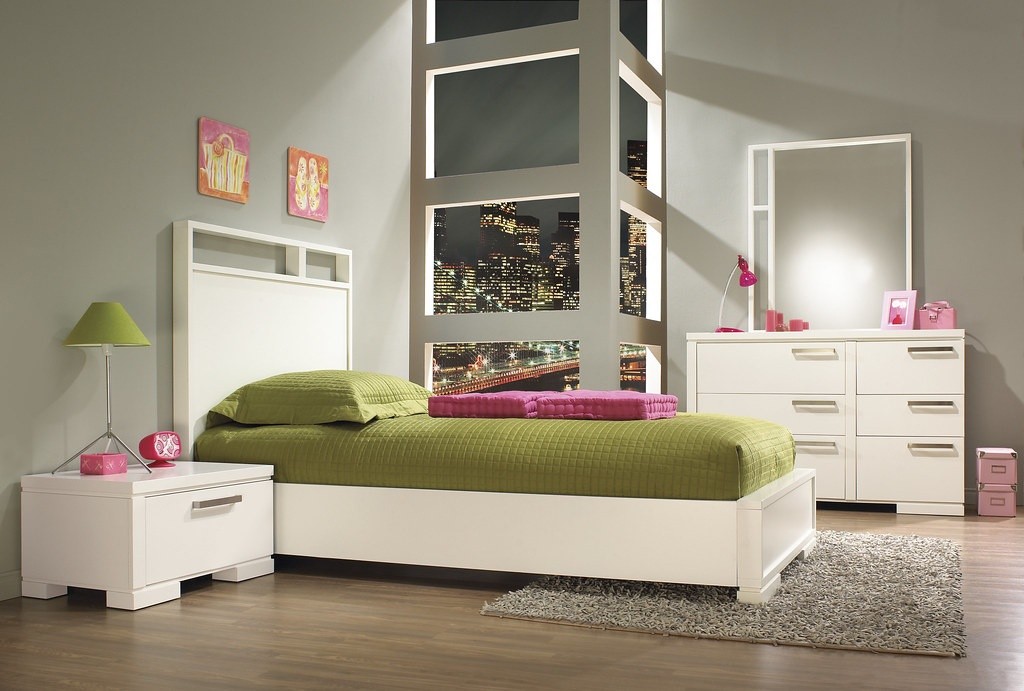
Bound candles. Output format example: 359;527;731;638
766;310;776;331
789;320;803;331
803;322;809;329
776;313;784;331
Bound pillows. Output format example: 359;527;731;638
205;369;438;426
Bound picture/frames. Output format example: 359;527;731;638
882;290;917;330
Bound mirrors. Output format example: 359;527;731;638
746;134;912;330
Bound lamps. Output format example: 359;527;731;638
715;254;757;332
51;300;154;476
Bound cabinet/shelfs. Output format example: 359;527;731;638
684;330;966;517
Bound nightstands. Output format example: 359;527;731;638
20;461;275;612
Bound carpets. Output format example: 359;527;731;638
477;528;968;660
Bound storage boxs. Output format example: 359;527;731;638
975;480;1017;517
914;301;957;329
976;447;1018;485
80;452;128;475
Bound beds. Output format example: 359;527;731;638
171;219;819;605
